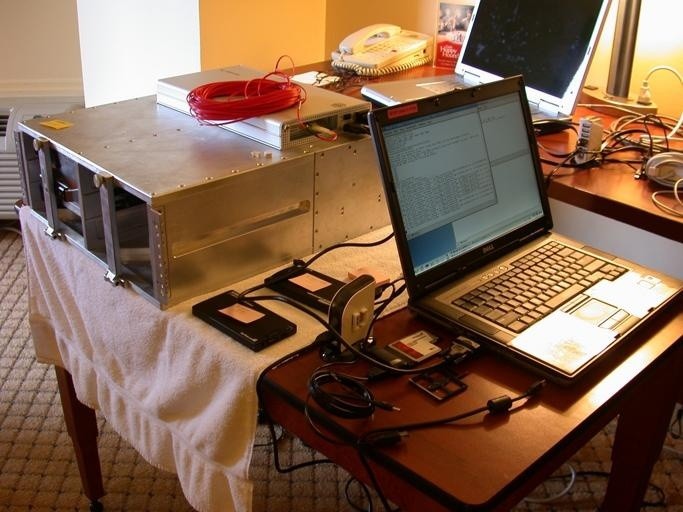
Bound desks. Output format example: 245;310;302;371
274;60;682;242
15;199;682;511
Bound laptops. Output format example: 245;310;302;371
361;72;682;390
359;0;612;124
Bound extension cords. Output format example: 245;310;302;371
580;84;659;119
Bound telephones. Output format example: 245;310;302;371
330;23;434;77
